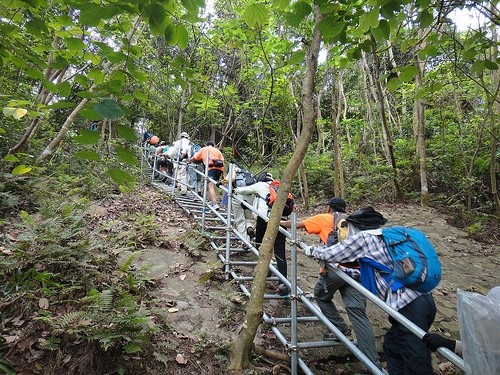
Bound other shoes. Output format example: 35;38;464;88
243;244;249;250
277;284;288;295
162;177;167;182
211;204;219;213
324;331;354;341
247;227;255;239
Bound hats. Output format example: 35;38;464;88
325;197;347;213
179;132;189;138
260;172;273;182
231;160;243;168
345;206;388;231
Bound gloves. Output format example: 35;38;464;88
422;332;456;353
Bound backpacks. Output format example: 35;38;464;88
265;181;295;216
326;212;360;267
190;144;203;163
359;225;441;301
235;169;257;186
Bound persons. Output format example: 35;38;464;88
179;142;203;201
230;172;289;296
140;131;181;190
216;159;255;250
163;132;194;195
422;330;462;357
187;141;225;213
291;207;436;374
280;198;389;375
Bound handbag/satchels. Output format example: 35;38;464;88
213;159;223;167
